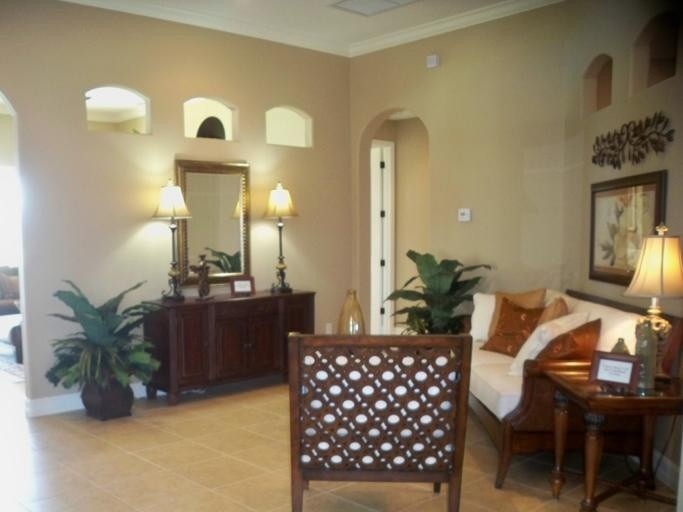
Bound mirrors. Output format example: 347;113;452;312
173;157;253;288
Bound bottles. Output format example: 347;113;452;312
635;321;656;397
338;288;365;335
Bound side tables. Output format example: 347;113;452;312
542;369;683;512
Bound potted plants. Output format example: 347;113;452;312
46;278;167;421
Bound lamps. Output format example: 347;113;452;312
262;181;303;294
149;176;194;302
620;220;683;389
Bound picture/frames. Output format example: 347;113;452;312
229;274;256;297
589;169;668;287
588;350;640;395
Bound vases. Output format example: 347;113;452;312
336;287;368;335
610;337;630;353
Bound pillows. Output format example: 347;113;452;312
478;296;568;358
505;311;588;378
533;316;602;361
466;288;547;345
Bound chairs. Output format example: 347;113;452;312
288;333;471;512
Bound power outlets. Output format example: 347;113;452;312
458;207;471;222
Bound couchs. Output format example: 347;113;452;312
467;288;683;491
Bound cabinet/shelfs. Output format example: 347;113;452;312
141;291;316;405
